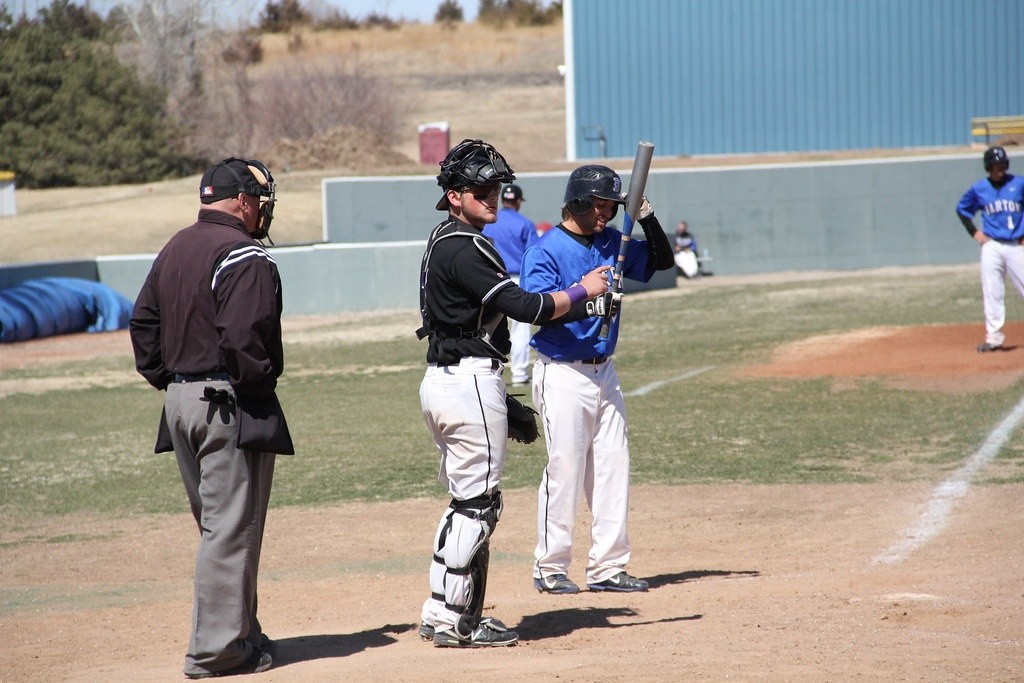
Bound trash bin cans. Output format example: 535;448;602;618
418;121;450;167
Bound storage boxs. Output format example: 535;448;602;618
417;119;449;165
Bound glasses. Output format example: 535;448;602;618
460;182;502;196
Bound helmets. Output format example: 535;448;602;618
564;164;623;214
983;147;1009;170
435;138;516;210
201;158;268;196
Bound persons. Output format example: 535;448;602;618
128;157;295;679
419;138;610;648
674;221;702;279
957;147;1024;353
482;185;539;388
518;164;675;594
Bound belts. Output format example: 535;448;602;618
437;359;503;374
552;356;607;364
171;372;229;380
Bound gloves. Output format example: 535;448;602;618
199;386;234;411
622;192;654;221
585;292;621;317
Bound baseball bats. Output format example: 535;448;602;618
609;143;655;316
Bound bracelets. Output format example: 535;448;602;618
562;283;588;311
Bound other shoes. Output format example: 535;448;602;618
976;343;999;352
185;652;272;678
512;375;530;384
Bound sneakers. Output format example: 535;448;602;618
588;570;648;592
420;616;507;640
534;573;580;594
433;624;519;647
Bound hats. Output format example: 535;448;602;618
501;185;524;202
